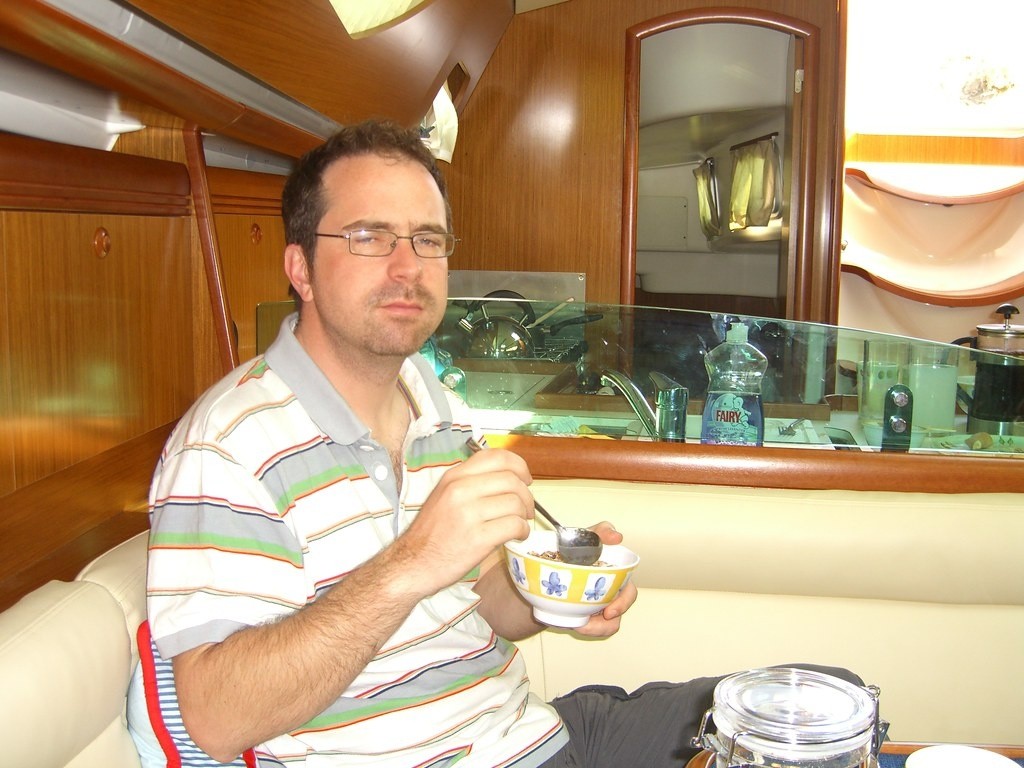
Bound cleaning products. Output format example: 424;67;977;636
700;323;768;446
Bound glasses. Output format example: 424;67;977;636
315;230;462;259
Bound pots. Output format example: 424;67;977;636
526;313;602;349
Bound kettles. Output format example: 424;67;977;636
941;303;1024;435
453;290;533;358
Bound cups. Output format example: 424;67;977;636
908;342;957;436
863;340;902;418
856;361;900;418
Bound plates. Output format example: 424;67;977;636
905;746;1024;768
931;435;1024;453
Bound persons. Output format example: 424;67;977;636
146;117;862;767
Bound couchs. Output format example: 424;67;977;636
0;427;1024;768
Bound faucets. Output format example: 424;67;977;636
600;365;688;442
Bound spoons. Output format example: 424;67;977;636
467;437;602;565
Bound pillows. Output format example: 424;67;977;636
125;620;284;768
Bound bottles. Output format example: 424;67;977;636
698;316;768;446
692;668;890;768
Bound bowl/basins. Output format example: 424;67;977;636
862;420;929;448
504;531;636;629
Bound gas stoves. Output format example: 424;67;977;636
532;337;592;374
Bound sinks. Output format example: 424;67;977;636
508;422;629;438
